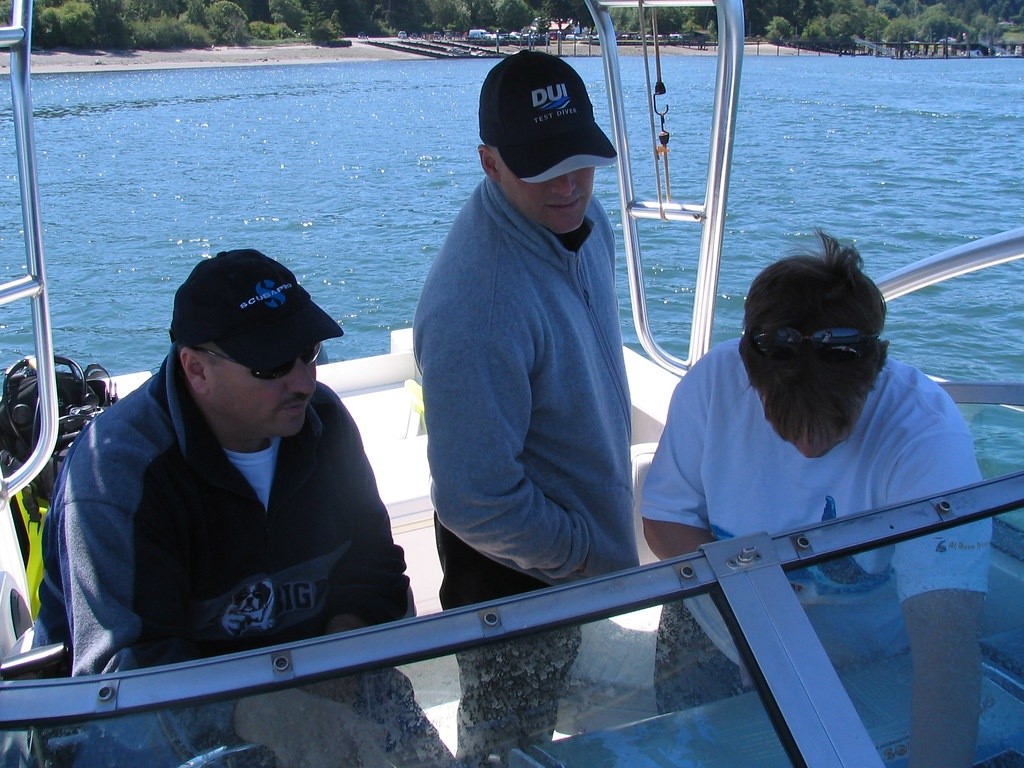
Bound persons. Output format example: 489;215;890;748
413;50;639;768
27;249;452;768
640;231;992;768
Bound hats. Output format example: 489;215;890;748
479;49;618;183
169;249;344;369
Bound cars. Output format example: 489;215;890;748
398;31;407;39
358;32;366;39
469;29;557;42
566;34;596;40
412;30;452;40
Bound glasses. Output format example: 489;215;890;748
742;325;879;364
191;342;321;379
